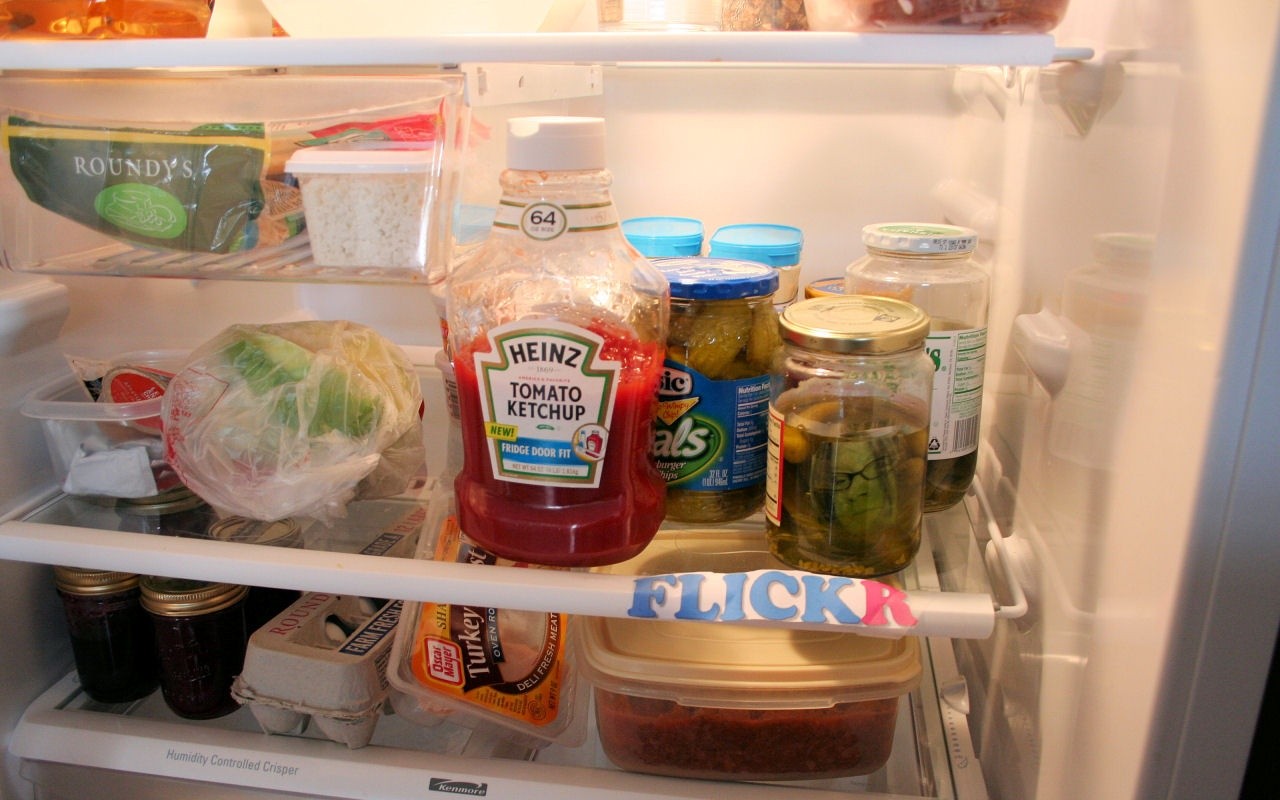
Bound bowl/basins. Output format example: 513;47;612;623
572;527;921;782
281;139;447;273
15;356;196;495
384;486;590;754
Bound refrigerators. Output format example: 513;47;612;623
0;0;1280;799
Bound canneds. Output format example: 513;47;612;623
613;216;987;580
52;484;304;721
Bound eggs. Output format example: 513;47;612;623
359;598;379;616
326;621;347;642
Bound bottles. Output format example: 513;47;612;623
51;496;307;722
444;115;672;565
